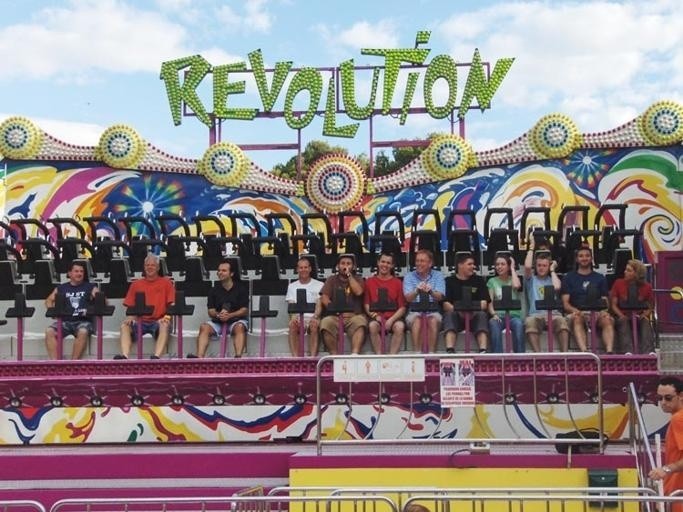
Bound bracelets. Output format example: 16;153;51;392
216;313;220;319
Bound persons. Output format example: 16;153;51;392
560;249;655;356
648;372;681;512
524;231;571;352
285;250;524;356
188;259;248;359
43;260;99;361
113;253;176;358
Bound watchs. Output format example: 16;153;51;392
661;463;672;478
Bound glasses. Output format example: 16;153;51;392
656;394;674;401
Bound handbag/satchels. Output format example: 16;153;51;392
555;428;609;455
